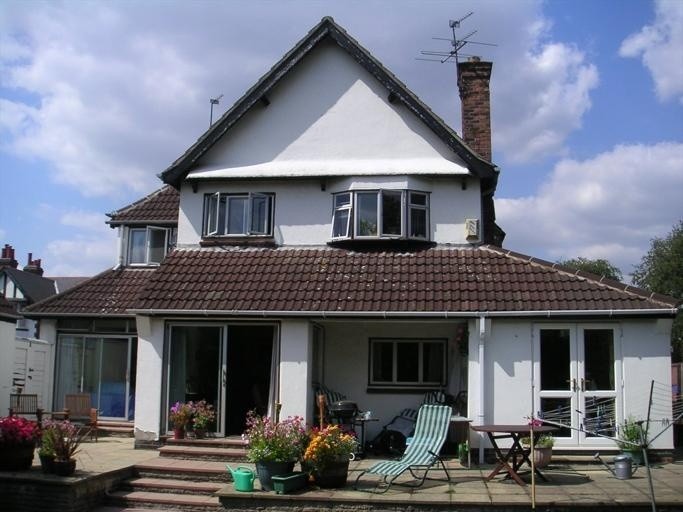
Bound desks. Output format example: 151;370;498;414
471;424;561;487
41;411;67;420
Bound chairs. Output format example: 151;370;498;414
63;393;98;442
353;405;452;494
8;393;43;430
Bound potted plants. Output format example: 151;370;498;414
192;399;216;438
49;422;96;476
615;414;649;464
521;434;554;469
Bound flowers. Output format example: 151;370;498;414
37;417;75;455
240;408;310;464
298;422;360;462
0;416;43;442
171;400;190;430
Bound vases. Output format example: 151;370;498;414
0;437;36;473
173;425;186;440
312;461;349;490
270;471;308;495
253;460;297;491
39;455;56;470
